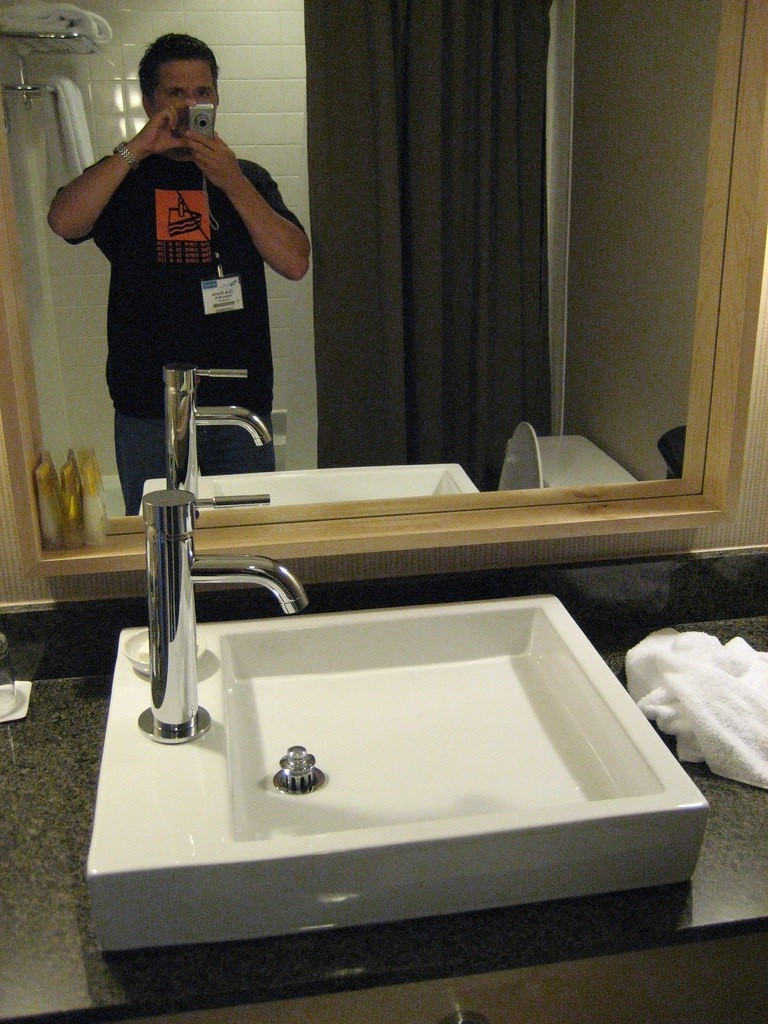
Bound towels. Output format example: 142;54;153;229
622;625;768;794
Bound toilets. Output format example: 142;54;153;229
496;420;640;492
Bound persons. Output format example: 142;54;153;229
45;32;311;515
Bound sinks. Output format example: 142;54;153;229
83;589;712;955
137;460;481;518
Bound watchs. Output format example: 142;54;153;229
113;142;138;168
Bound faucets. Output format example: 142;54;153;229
137;487;311;745
160;360;273;501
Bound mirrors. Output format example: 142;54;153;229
0;0;764;581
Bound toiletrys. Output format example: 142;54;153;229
32;446;107;517
34;454;107;553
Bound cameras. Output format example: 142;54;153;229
189;103;215;139
